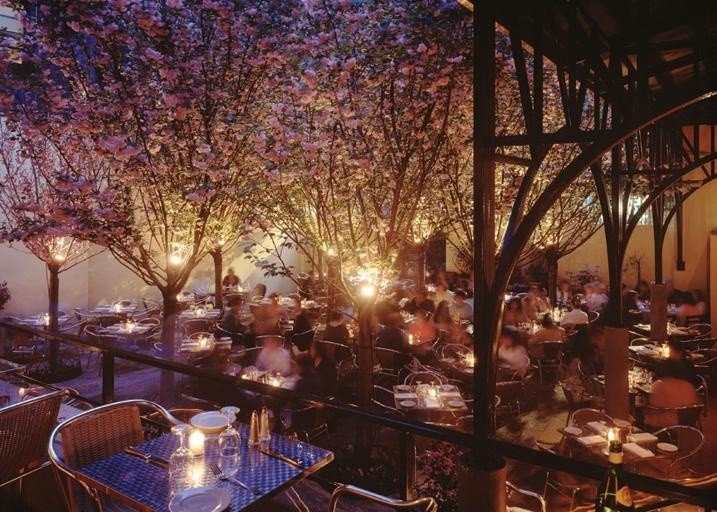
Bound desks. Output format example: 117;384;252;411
629;308;679;322
628;344;704;363
556;425;675;469
97;323;157;352
89;307;138;318
633;325;703;341
18;315;74;354
226;367;297;405
75;421;334;512
519;325;578;364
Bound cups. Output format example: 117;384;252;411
218;406;242;457
169;424;194;481
415;381;423;394
624;424;635;444
428;381;437;396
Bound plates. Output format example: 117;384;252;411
168;486;231;511
446;400;464;407
440;384;454;390
189;411;236;433
602;448;623;456
396;385;410;389
399;400;416;407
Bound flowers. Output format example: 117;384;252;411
416;443;465;503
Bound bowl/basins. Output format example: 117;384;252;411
612;418;631;427
656;442;678;451
563;426;582;434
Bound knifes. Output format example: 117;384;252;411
251;443;304;467
123;446;169;470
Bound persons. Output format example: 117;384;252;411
401;289;434;320
534;313;566;342
324;310;349;346
375;313;408;353
433;281;454;304
560;298;588;325
498;326;529;377
502;297;531;326
297;272;314;300
667;289;681;305
222;267;244;292
622;283;638;311
285;295;314;352
634;280;650;300
656;341;702;426
459;280;473;299
676;291;699;326
254;332;291;378
522;286;551;321
219;294;248;334
691;289;706;315
408;309;435;356
242;294;289;348
554;279;608;311
429;301;455;333
448;288;473;324
351;287;401;321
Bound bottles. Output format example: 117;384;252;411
594;439;634;511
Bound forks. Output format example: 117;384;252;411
209;463;262;497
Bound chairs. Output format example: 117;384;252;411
588;311;600;327
404;372;442;385
291;324;320;356
58;310;65;315
653;426;705;474
216;323;243;344
629;331;648;345
224;294;245;305
279;395;335;444
375;347;413;384
176;302;187;312
112;300;131;306
0;389;71;512
371;324;384;342
58;319;88;365
415;423;457;454
688;324;712;338
634;404;704;434
456;395;501;436
414;357;448;385
315;340;359;382
154;343;180;357
195;296;210;305
629;338;656;346
183;320;211;337
85;325;127;377
94;316;120;327
506;482;546;512
189;332;210;339
628;349;649;367
531;341;564;384
138;318;160;355
194;292;206;304
680;339;717;351
572;409;614;426
228;347;264;367
535;442;597;512
257;359;298;376
408;335;440;362
685;314;707;327
73;308;96;337
0;317;38;351
578;362;604;401
511;366;539;405
328;485;438;512
252;283;266;300
256;335;284;347
160;312;185;347
496;381;522;435
369;385;404;436
691;349;717;417
442;343;472;359
48;399;184;512
559;382;593;426
143;298;160;318
